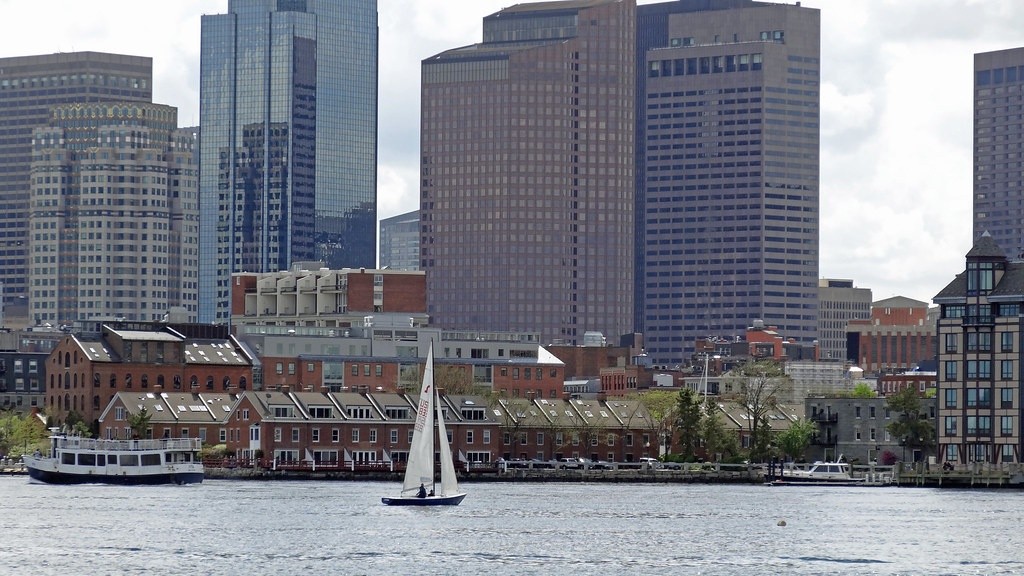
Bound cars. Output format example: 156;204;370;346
593;461;614;470
553;458;578;469
576;457;594;469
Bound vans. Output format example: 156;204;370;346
639;458;660;469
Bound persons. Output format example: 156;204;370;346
428;490;435;497
133;432;140;450
35;448;42;458
161;432;171;449
417;483;426;498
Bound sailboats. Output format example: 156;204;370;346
380;336;468;505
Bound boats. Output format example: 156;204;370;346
21;437;204;484
764;462;866;486
